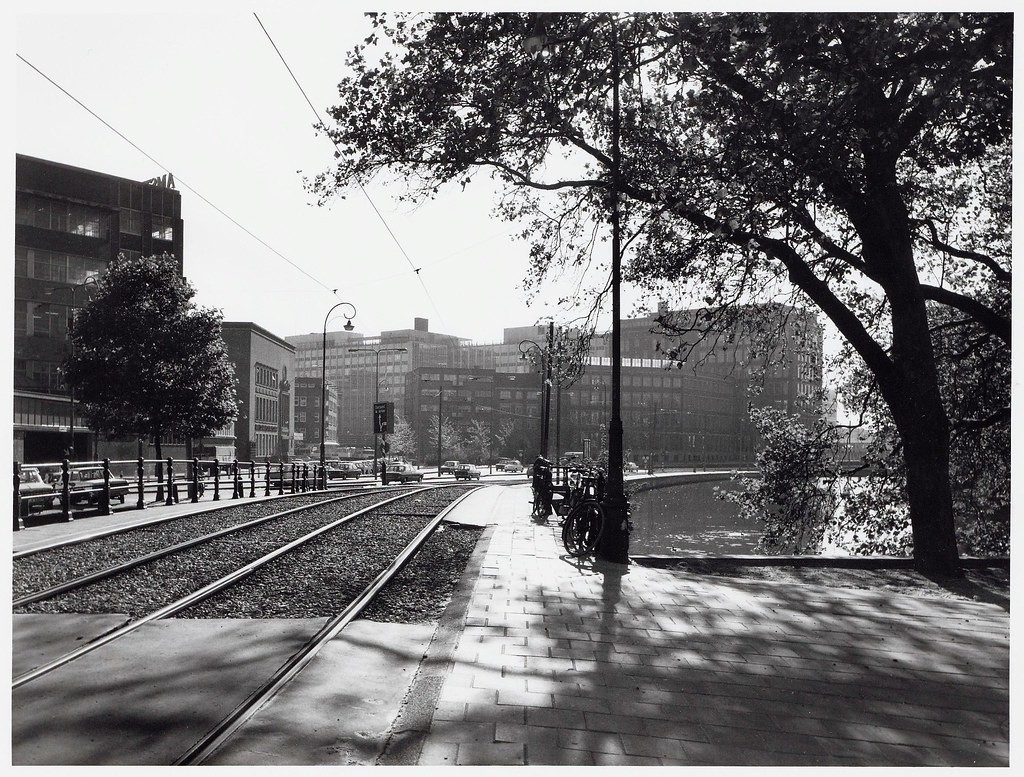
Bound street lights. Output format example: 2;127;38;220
468;374;516;474
421;379;441;477
344;347;408;480
518;322;554;456
45;276;105;462
319;302;356;490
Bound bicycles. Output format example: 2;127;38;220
527;457;609;557
198;475;206;498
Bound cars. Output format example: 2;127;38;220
504;461;524;472
264;464;321;488
496;458;512;471
328;463;362;480
19;468;55;515
308;456;412;475
380;465;424;484
52;467;131;505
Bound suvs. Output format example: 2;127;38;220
441;461;460;475
454;464;481;480
